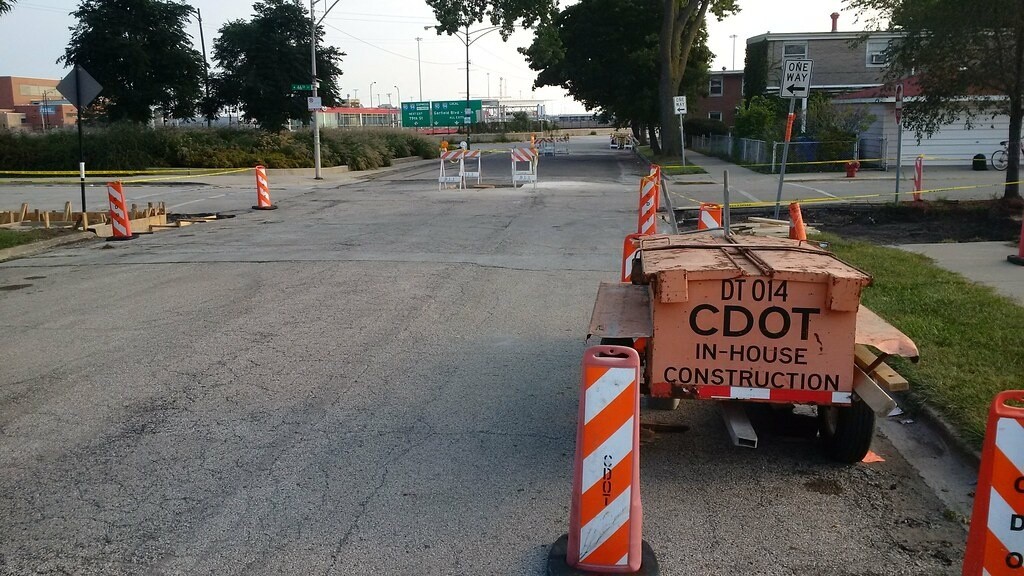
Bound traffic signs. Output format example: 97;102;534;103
673;96;687;114
402;100;483;127
779;58;814;98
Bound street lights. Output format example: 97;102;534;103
424;25;538;150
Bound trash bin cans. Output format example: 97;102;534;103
795;137;819;163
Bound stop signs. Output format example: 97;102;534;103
896;84;903;125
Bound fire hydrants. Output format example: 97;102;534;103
845;161;860;177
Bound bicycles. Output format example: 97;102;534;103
990;136;1024;172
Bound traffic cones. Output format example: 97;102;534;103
1008;215;1024;266
